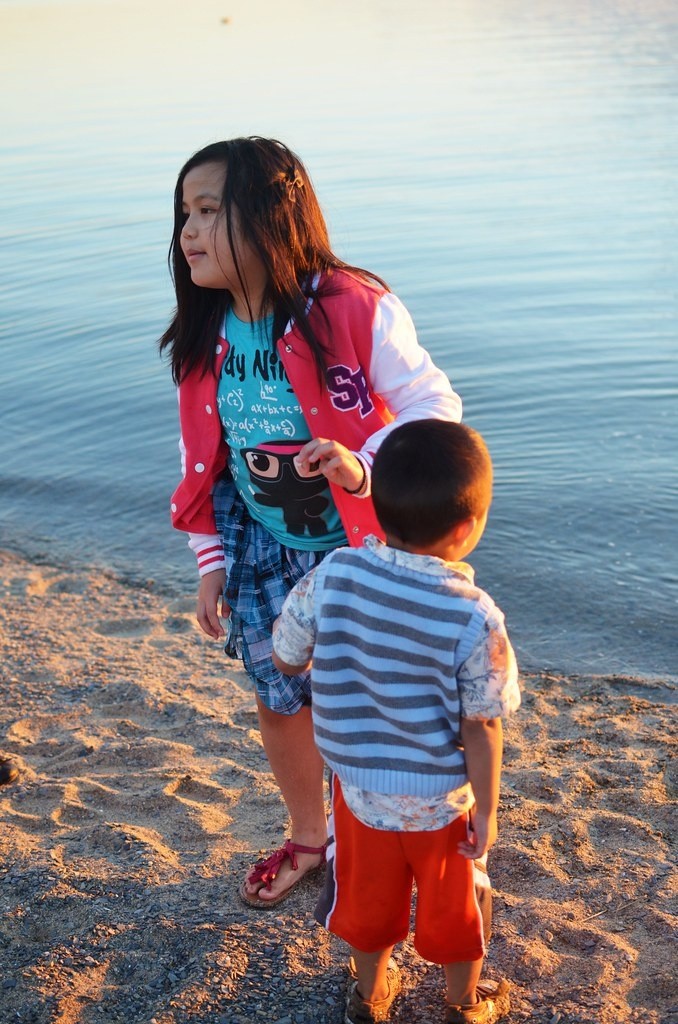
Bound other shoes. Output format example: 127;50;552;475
344;956;402;1024
445;979;510;1024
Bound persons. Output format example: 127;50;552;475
159;138;465;909
271;419;523;1023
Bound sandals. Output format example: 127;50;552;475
239;838;327;906
